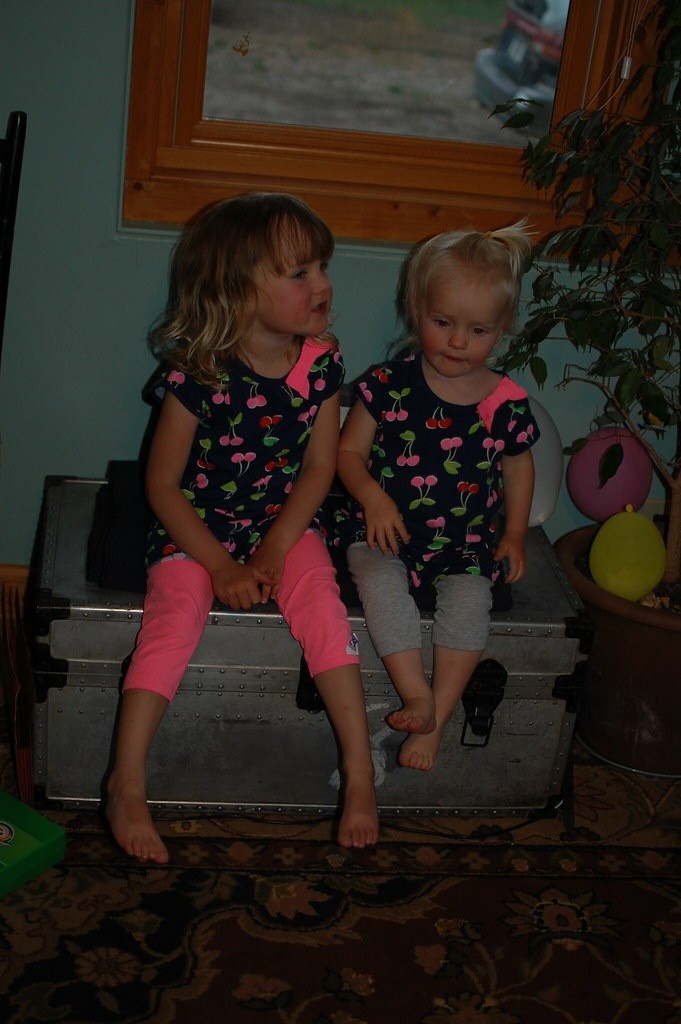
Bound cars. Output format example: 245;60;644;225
472;0;570;146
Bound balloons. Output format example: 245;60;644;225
527;395;665;602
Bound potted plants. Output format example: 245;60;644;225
488;0;681;783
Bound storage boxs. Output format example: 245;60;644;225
21;473;603;821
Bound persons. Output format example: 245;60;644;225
106;195;379;863
334;220;540;771
